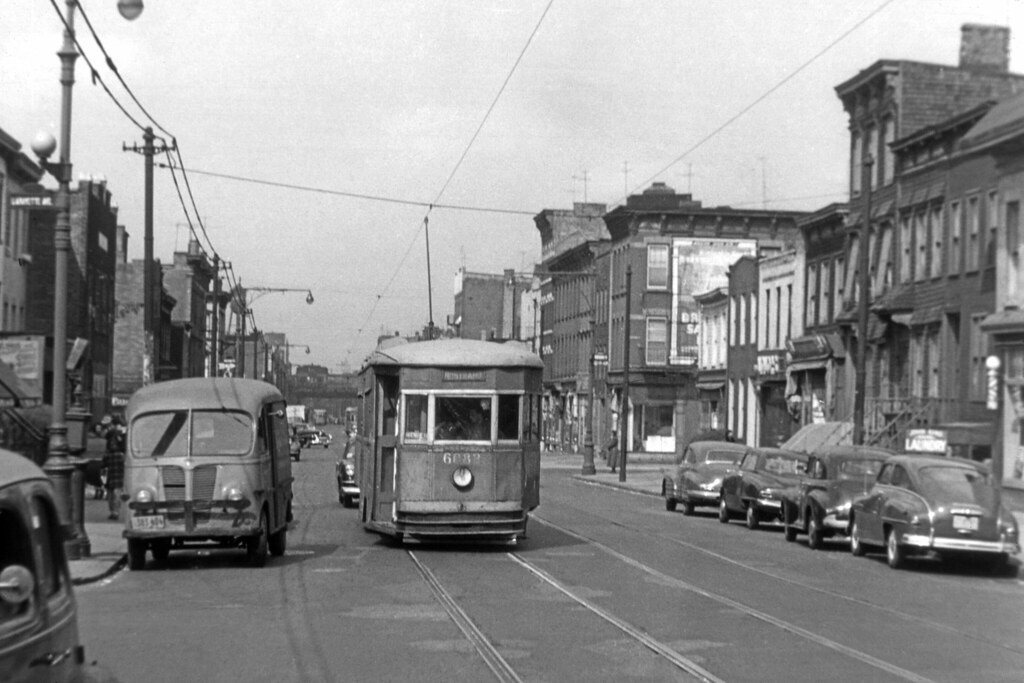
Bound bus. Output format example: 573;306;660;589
360;337;545;545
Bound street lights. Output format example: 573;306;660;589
507;269;598;473
42;0;144;560
272;343;310;382
238;288;314;374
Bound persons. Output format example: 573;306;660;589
465;405;505;440
100;416;126;519
605;429;618;474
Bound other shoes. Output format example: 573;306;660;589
108;514;117;520
94;490;103;498
610;470;616;474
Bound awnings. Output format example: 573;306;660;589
867;281;915;312
981;311;1024;337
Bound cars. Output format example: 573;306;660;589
779;444;901;549
287;404;333;459
0;445;79;682
336;438;361;508
845;455;1017;575
663;442;748;514
718;448;810;528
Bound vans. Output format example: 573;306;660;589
119;379;292;568
